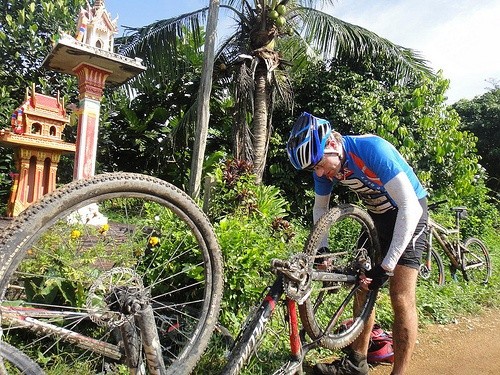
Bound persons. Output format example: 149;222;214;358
287;112;428;375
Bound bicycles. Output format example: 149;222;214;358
416;199;492;288
0;172;383;375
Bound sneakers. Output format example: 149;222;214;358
313;355;369;375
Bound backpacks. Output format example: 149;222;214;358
334;318;395;364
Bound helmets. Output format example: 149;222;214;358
287;112;331;172
